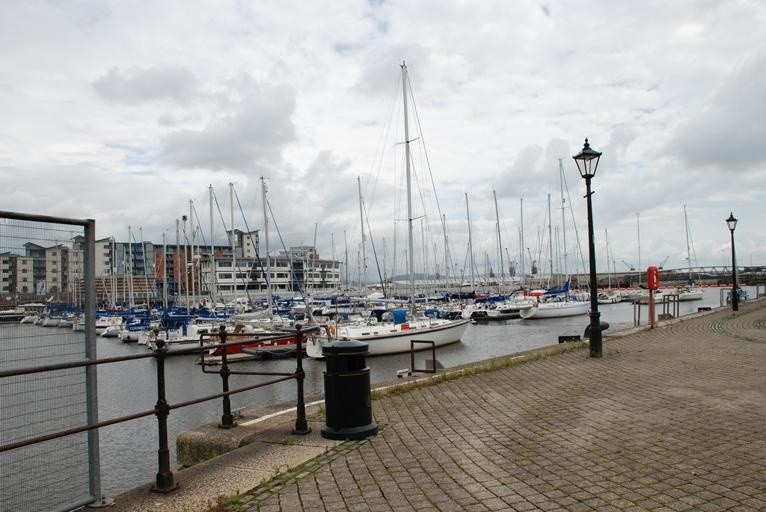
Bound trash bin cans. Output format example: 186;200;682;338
319;337;378;440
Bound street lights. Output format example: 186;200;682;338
725;211;740;312
